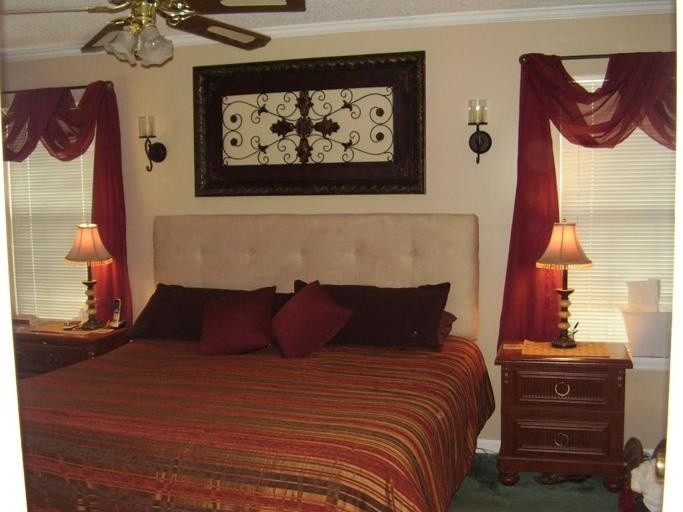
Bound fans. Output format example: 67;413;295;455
77;1;307;55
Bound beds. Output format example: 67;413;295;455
13;211;480;511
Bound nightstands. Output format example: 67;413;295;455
13;319;127;379
495;339;634;492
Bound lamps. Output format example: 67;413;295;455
64;222;114;330
136;115;167;173
104;15;173;68
532;217;594;349
465;98;491;164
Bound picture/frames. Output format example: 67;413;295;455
192;50;426;196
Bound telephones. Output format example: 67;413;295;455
110;298;126;328
